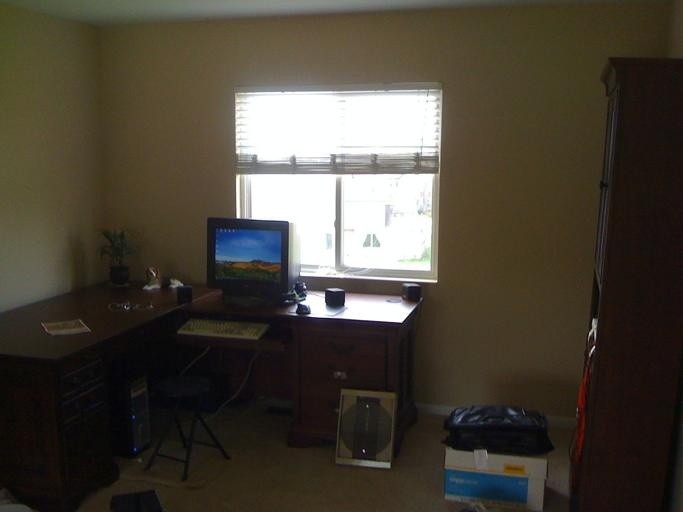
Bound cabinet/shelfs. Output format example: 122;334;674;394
568;58;683;511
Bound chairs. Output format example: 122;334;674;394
134;332;232;483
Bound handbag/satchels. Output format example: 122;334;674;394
440;403;554;457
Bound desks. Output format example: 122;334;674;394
1;274;421;511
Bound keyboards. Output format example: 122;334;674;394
177;314;271;340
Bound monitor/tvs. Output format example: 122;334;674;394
206;216;288;308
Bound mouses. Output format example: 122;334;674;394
296;302;310;314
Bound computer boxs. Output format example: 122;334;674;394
110;369;153;457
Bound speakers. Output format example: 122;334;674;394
325;287;345;308
177;285;192;304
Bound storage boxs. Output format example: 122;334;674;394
440;446;549;511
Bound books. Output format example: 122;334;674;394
41;318;92;337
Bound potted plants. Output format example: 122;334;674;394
102;230;132;285
